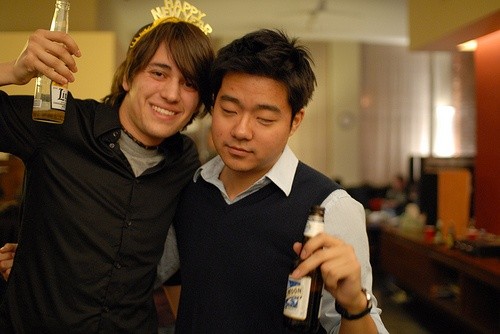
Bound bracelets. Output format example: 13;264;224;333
334;287;373;321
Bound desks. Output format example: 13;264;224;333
367;213;500;334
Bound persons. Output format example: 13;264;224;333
1;0;221;333
0;28;390;334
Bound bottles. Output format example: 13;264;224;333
282;205;326;334
32;1;70;125
